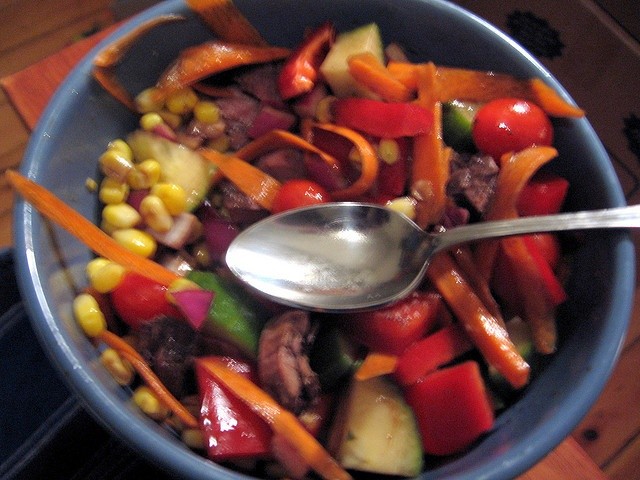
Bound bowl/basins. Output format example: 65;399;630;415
13;0;638;480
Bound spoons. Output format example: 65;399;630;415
224;200;639;312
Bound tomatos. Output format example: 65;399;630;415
270;179;334;216
112;269;184;332
473;99;553;159
